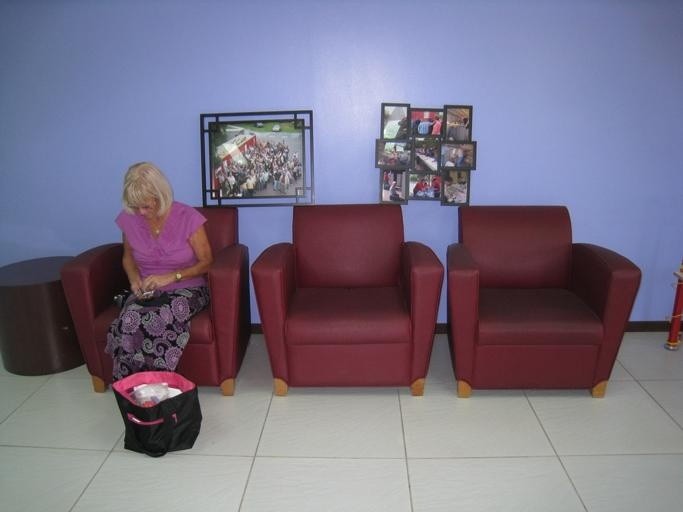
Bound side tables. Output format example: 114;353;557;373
1;256;87;376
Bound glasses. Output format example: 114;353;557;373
136;287;155;299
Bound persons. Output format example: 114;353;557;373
104;162;214;383
216;134;302;197
383;115;469;201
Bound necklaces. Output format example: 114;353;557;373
150;219;164;234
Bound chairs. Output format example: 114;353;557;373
249;203;445;397
446;206;641;398
58;206;249;396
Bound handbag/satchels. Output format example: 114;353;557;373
112;369;202;458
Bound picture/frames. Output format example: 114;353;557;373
200;110;315;207
375;103;476;206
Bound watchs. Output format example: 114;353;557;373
175;271;182;281
